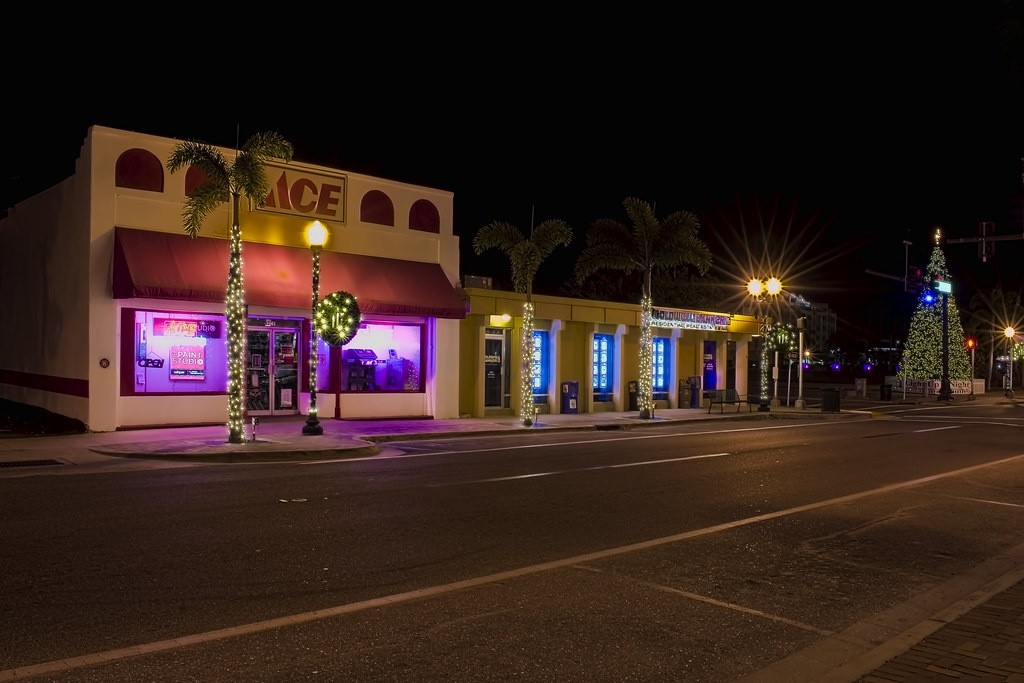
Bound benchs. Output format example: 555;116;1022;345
704;389;753;415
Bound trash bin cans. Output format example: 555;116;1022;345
562;381;578;414
820;388;840;413
879;384;893;401
628;381;639;410
680;376;692;408
687;376;701;408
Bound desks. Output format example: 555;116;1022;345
347;362;376;391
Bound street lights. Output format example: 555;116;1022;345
746;278;782;412
302;219;328;437
1005;327;1015;398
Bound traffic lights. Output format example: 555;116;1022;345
968;339;974;347
